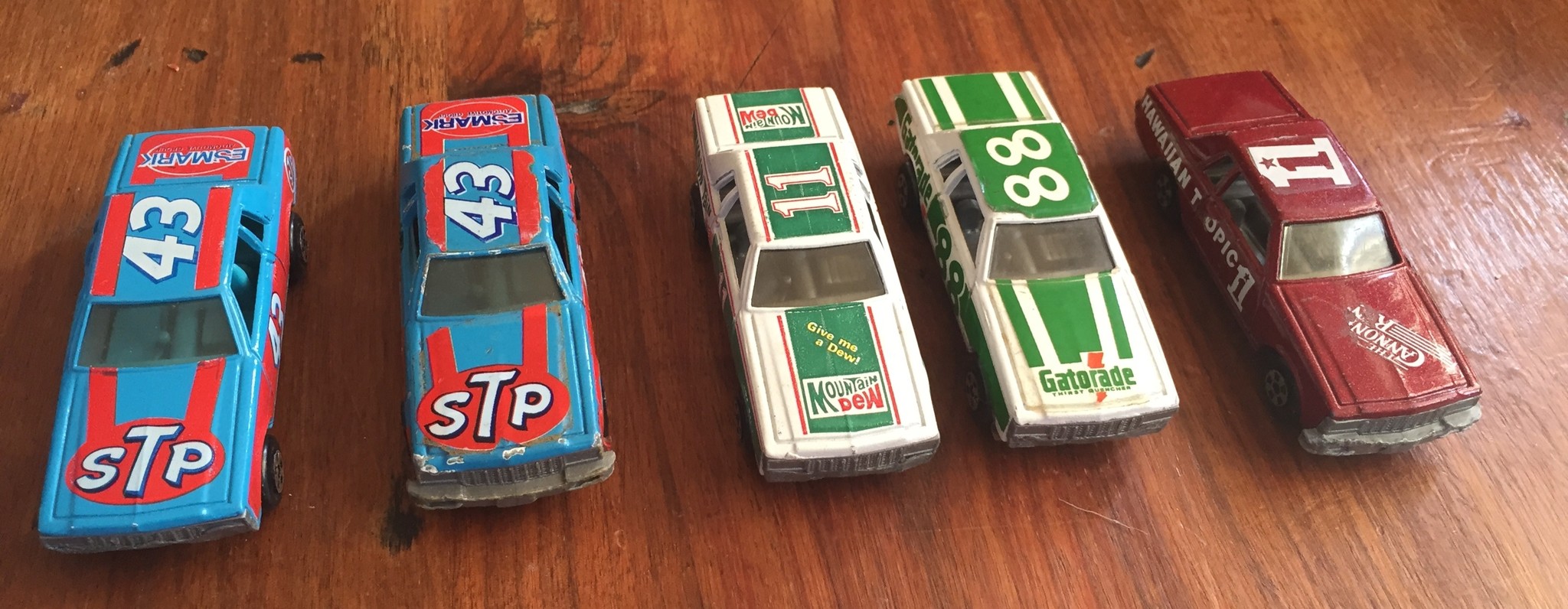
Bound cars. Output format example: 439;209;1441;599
395;91;618;510
893;70;1180;451
692;84;941;485
1133;70;1483;457
38;125;307;554
